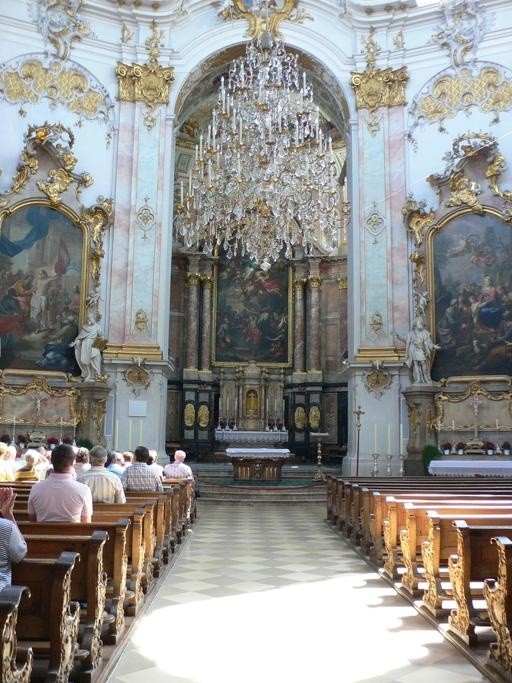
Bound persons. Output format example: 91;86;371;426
218;269;287;362
1;486;29;587
29;443;94;523
0;441;194;490
79;445;128;503
0;266;105;381
394;274;512;383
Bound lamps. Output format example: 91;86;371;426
174;21;346;280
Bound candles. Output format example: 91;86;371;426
13;414;80;429
437;417;503;433
113;418;145;453
373;422;405;453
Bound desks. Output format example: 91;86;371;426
225;445;291;485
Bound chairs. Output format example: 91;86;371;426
323;474;512;683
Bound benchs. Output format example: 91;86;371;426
0;469;195;683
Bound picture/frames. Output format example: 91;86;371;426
0;191;117;379
416;200;512;381
208;245;297;370
